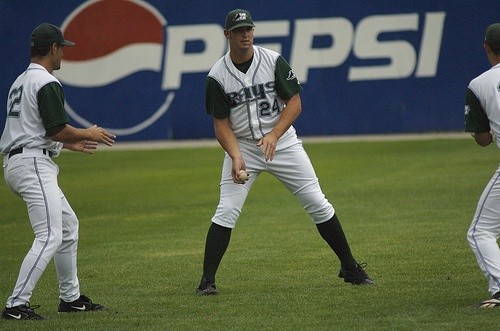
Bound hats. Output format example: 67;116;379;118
225;9;255;32
481;23;500;48
30;22;76;46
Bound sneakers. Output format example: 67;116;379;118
478;291;500;309
2;301;45;320
197;280;218;295
338;262;369;286
58;294;106;312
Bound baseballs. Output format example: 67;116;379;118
235;169;247;182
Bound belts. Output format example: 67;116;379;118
9;147;53;157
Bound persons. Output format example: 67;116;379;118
195;9;372;294
0;23;116;319
464;23;500;310
